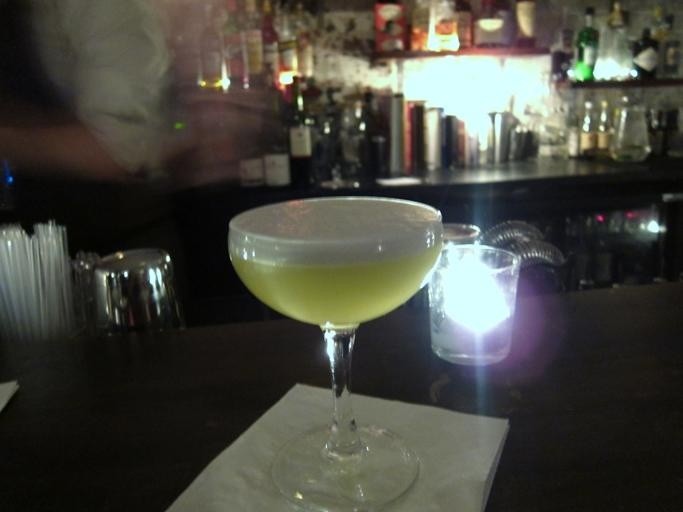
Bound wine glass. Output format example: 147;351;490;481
228;196;443;507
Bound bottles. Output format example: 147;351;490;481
564;87;667;165
177;0;314;98
554;0;683;85
237;72;392;187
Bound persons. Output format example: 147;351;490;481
1;0;204;330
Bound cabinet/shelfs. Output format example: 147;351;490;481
371;46;683;90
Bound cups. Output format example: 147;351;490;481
93;248;182;329
428;243;521;366
